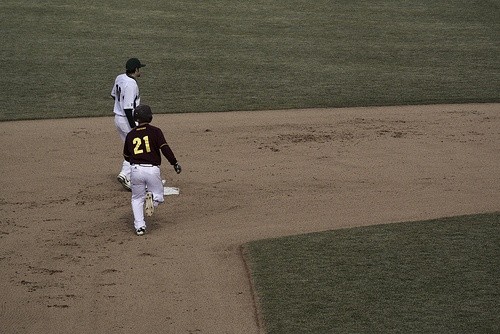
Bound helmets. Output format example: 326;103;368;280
133;105;152;122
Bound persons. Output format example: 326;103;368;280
123;105;182;236
110;58;148;192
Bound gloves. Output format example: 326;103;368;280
174;164;182;174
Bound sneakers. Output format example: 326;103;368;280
145;192;154;217
116;173;132;192
137;226;146;235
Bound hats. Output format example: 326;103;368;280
126;58;146;70
